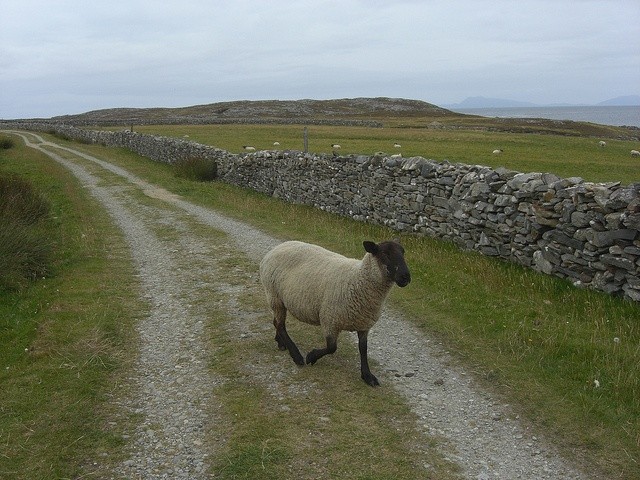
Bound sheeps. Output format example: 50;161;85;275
261;240;412;387
